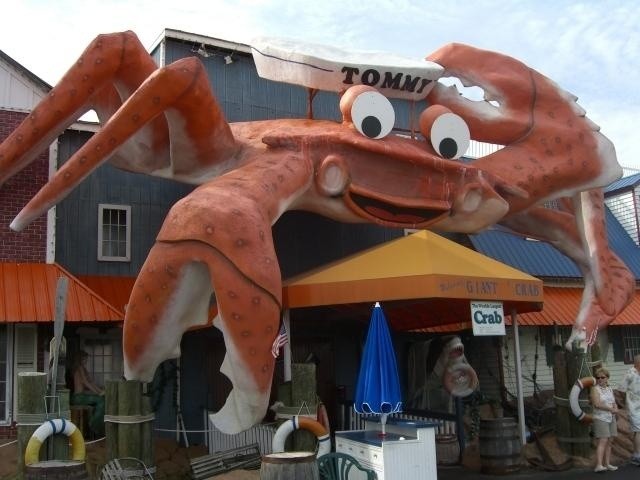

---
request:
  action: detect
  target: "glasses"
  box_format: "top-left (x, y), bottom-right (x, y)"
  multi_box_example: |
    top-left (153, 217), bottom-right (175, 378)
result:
top-left (596, 377), bottom-right (608, 379)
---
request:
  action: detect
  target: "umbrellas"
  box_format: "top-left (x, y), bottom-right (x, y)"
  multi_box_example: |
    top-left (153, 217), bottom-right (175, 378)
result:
top-left (353, 301), bottom-right (404, 436)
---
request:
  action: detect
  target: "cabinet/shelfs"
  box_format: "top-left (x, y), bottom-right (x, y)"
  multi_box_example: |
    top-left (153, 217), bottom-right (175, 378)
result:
top-left (335, 428), bottom-right (440, 479)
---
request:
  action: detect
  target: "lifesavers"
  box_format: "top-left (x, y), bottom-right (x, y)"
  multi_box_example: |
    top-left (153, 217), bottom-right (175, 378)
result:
top-left (272, 416), bottom-right (332, 466)
top-left (569, 377), bottom-right (598, 423)
top-left (24, 418), bottom-right (86, 466)
top-left (442, 363), bottom-right (478, 397)
top-left (319, 404), bottom-right (331, 439)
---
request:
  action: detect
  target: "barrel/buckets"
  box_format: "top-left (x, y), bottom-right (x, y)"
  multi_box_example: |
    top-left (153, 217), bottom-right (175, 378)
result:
top-left (24, 460), bottom-right (90, 480)
top-left (436, 433), bottom-right (460, 465)
top-left (478, 417), bottom-right (521, 475)
top-left (258, 451), bottom-right (321, 480)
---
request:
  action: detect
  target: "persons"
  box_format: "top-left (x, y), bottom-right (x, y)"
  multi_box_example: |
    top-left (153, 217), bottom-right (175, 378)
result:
top-left (69, 350), bottom-right (105, 436)
top-left (616, 355), bottom-right (640, 466)
top-left (589, 367), bottom-right (618, 473)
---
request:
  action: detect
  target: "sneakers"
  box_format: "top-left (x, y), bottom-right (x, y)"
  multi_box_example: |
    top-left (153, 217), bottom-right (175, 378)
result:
top-left (630, 455), bottom-right (640, 465)
top-left (604, 465), bottom-right (618, 470)
top-left (594, 466), bottom-right (607, 472)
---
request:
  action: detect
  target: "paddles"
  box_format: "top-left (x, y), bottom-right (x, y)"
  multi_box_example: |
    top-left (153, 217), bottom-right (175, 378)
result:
top-left (48, 277), bottom-right (68, 459)
